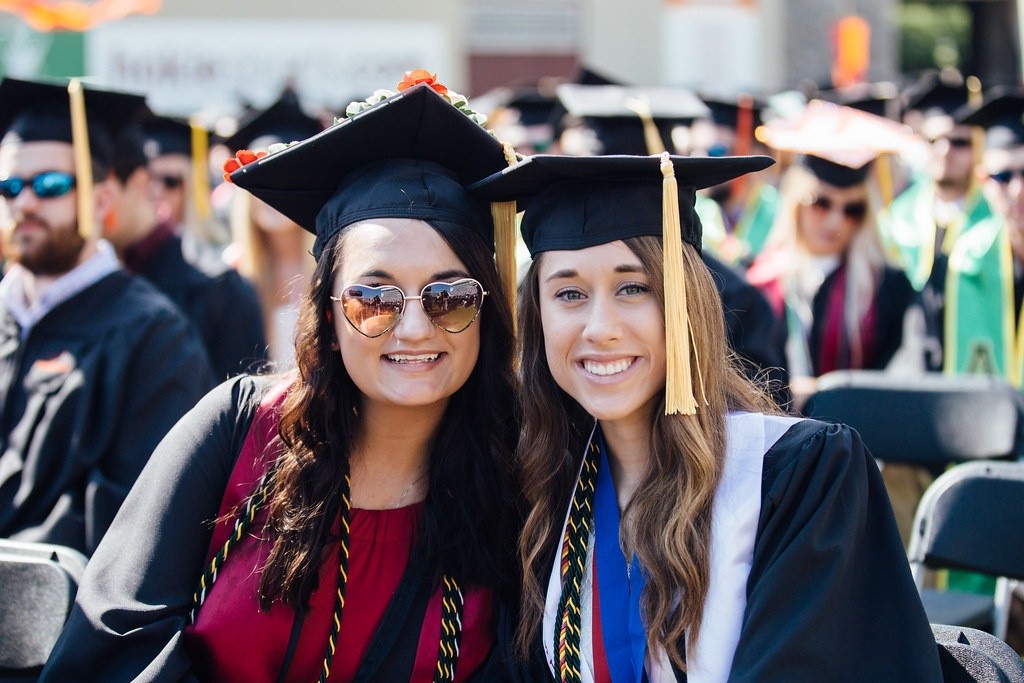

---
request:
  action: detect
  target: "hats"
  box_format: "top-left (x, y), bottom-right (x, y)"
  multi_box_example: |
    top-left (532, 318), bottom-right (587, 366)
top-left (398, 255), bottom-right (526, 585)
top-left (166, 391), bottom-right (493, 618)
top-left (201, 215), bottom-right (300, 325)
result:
top-left (466, 152), bottom-right (777, 417)
top-left (151, 102), bottom-right (317, 152)
top-left (230, 84), bottom-right (533, 381)
top-left (0, 78), bottom-right (146, 240)
top-left (484, 69), bottom-right (1024, 219)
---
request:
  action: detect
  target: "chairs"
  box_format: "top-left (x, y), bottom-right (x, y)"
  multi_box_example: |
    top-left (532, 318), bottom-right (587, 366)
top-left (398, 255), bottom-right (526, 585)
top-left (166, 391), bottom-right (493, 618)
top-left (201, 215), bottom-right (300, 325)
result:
top-left (1, 542), bottom-right (90, 677)
top-left (797, 367), bottom-right (1023, 683)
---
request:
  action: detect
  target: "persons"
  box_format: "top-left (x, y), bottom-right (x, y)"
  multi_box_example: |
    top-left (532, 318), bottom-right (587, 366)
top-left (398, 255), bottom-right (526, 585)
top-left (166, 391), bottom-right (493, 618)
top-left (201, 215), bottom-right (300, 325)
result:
top-left (0, 72), bottom-right (1024, 601)
top-left (36, 85), bottom-right (547, 683)
top-left (472, 152), bottom-right (945, 683)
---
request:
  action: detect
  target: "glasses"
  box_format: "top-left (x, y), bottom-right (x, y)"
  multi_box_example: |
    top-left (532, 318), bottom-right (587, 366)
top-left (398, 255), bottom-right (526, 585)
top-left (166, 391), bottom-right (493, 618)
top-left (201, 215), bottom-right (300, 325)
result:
top-left (152, 173), bottom-right (184, 192)
top-left (925, 137), bottom-right (971, 148)
top-left (987, 169), bottom-right (1024, 183)
top-left (0, 173), bottom-right (76, 202)
top-left (329, 277), bottom-right (489, 338)
top-left (803, 194), bottom-right (866, 223)
